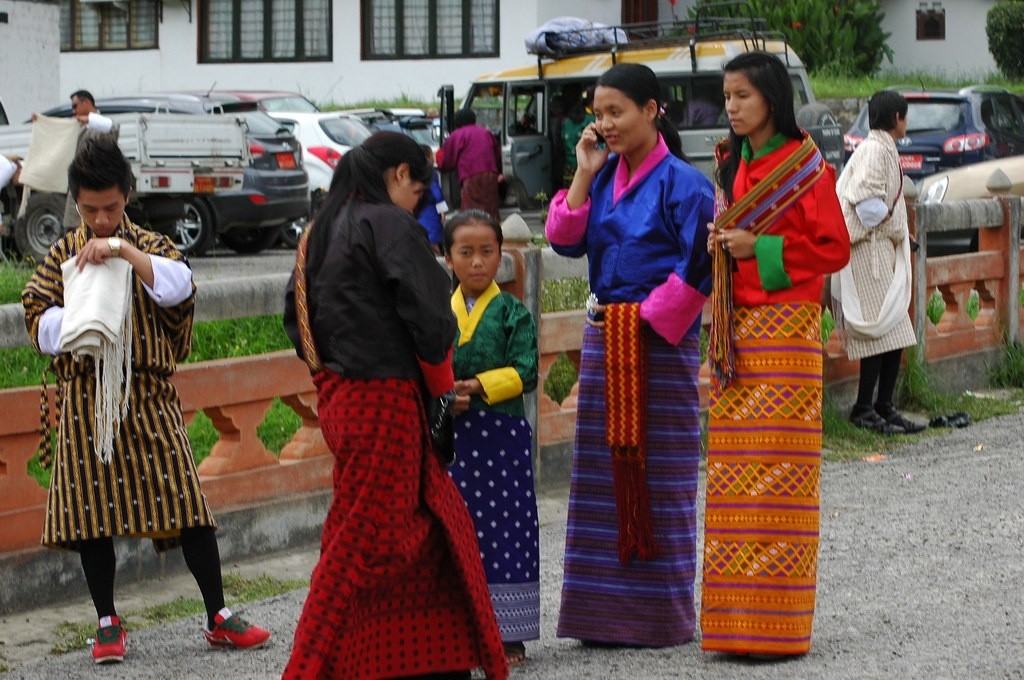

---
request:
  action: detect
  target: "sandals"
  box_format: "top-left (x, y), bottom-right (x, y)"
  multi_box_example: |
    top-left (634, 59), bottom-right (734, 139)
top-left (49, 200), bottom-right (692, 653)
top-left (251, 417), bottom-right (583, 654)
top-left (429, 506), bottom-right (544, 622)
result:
top-left (503, 641), bottom-right (525, 664)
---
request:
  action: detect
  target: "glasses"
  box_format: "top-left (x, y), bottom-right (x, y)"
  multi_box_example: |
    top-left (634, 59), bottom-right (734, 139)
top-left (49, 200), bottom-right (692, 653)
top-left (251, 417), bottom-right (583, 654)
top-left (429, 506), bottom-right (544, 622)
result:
top-left (71, 101), bottom-right (82, 110)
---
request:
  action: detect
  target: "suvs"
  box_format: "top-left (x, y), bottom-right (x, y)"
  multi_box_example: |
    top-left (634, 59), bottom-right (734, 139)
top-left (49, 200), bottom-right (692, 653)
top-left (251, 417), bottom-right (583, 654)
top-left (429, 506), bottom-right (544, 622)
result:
top-left (843, 83), bottom-right (1024, 184)
top-left (41, 93), bottom-right (310, 257)
top-left (432, 2), bottom-right (847, 210)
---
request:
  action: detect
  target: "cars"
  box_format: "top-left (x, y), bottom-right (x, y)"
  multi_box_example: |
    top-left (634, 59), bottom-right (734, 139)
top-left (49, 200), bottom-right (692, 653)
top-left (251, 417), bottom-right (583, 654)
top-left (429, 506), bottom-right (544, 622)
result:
top-left (915, 156), bottom-right (1024, 253)
top-left (232, 93), bottom-right (447, 249)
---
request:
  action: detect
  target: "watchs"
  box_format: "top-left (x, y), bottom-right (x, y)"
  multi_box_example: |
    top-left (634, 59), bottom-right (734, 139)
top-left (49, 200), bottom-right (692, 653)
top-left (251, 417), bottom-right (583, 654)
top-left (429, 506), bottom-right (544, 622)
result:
top-left (108, 237), bottom-right (120, 257)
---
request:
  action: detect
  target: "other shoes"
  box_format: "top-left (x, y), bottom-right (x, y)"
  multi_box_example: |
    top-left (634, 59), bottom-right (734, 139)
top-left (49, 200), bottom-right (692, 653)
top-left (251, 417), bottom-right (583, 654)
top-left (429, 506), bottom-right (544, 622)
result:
top-left (848, 404), bottom-right (904, 433)
top-left (874, 402), bottom-right (927, 431)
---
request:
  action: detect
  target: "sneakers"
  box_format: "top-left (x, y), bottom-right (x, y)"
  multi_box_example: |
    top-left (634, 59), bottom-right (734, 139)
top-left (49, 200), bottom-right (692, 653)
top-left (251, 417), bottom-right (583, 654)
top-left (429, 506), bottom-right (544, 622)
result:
top-left (92, 615), bottom-right (128, 663)
top-left (205, 607), bottom-right (271, 649)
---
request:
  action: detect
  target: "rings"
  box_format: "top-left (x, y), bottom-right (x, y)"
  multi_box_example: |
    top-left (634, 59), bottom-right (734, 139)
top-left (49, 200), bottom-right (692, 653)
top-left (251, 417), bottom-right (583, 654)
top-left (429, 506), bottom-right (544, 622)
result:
top-left (722, 243), bottom-right (726, 249)
top-left (722, 233), bottom-right (725, 241)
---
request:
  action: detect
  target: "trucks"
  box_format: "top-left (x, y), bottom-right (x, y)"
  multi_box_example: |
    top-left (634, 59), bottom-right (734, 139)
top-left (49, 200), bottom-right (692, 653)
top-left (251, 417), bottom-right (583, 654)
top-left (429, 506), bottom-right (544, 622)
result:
top-left (0, 95), bottom-right (248, 264)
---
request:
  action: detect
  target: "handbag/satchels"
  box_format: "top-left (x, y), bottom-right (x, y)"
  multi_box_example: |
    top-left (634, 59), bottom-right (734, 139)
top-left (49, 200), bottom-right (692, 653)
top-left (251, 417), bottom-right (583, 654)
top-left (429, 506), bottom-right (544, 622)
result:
top-left (525, 17), bottom-right (627, 52)
top-left (421, 385), bottom-right (455, 465)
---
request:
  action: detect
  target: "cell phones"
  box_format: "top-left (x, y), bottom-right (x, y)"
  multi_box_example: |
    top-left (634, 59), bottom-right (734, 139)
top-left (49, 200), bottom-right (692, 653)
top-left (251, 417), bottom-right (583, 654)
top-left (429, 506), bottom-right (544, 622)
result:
top-left (595, 130), bottom-right (605, 149)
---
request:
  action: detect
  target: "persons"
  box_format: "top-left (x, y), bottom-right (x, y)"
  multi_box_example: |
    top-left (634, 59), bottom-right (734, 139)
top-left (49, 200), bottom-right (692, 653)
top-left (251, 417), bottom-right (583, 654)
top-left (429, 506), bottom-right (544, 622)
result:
top-left (0, 152), bottom-right (22, 264)
top-left (436, 108), bottom-right (504, 223)
top-left (545, 63), bottom-right (716, 647)
top-left (831, 91), bottom-right (926, 436)
top-left (31, 89), bottom-right (120, 233)
top-left (699, 51), bottom-right (851, 655)
top-left (666, 98), bottom-right (720, 129)
top-left (565, 85), bottom-right (605, 158)
top-left (20, 132), bottom-right (272, 664)
top-left (282, 130), bottom-right (541, 680)
